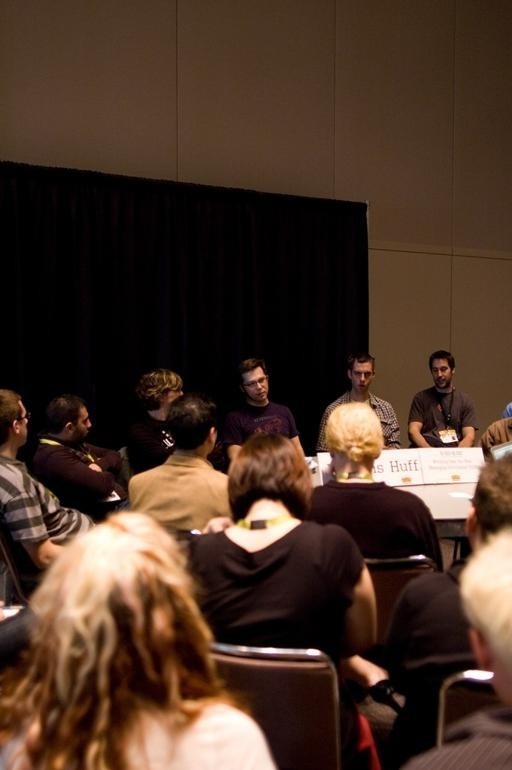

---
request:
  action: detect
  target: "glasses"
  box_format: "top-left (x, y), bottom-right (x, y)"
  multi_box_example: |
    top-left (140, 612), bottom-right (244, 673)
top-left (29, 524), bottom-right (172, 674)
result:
top-left (243, 376), bottom-right (266, 387)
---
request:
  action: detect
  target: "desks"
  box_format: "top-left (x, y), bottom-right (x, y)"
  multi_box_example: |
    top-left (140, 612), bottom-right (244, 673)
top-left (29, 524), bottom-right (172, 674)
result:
top-left (381, 478), bottom-right (476, 574)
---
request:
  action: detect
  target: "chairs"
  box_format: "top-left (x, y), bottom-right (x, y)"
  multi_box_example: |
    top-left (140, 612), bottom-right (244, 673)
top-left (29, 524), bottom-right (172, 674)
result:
top-left (438, 669), bottom-right (495, 741)
top-left (207, 642), bottom-right (341, 770)
top-left (363, 556), bottom-right (437, 647)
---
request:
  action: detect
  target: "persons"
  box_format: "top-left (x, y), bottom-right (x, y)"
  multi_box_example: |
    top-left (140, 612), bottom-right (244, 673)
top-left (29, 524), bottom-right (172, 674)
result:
top-left (30, 390), bottom-right (132, 518)
top-left (125, 366), bottom-right (188, 477)
top-left (181, 427), bottom-right (407, 769)
top-left (388, 452), bottom-right (512, 699)
top-left (300, 401), bottom-right (445, 577)
top-left (1, 387), bottom-right (101, 569)
top-left (316, 355), bottom-right (402, 455)
top-left (223, 354), bottom-right (307, 473)
top-left (479, 401), bottom-right (512, 461)
top-left (129, 394), bottom-right (232, 544)
top-left (0, 509), bottom-right (276, 770)
top-left (396, 525), bottom-right (512, 768)
top-left (407, 351), bottom-right (479, 451)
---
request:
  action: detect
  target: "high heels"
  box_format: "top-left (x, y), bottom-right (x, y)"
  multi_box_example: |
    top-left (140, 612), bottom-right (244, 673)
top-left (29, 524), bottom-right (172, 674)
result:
top-left (365, 679), bottom-right (404, 716)
